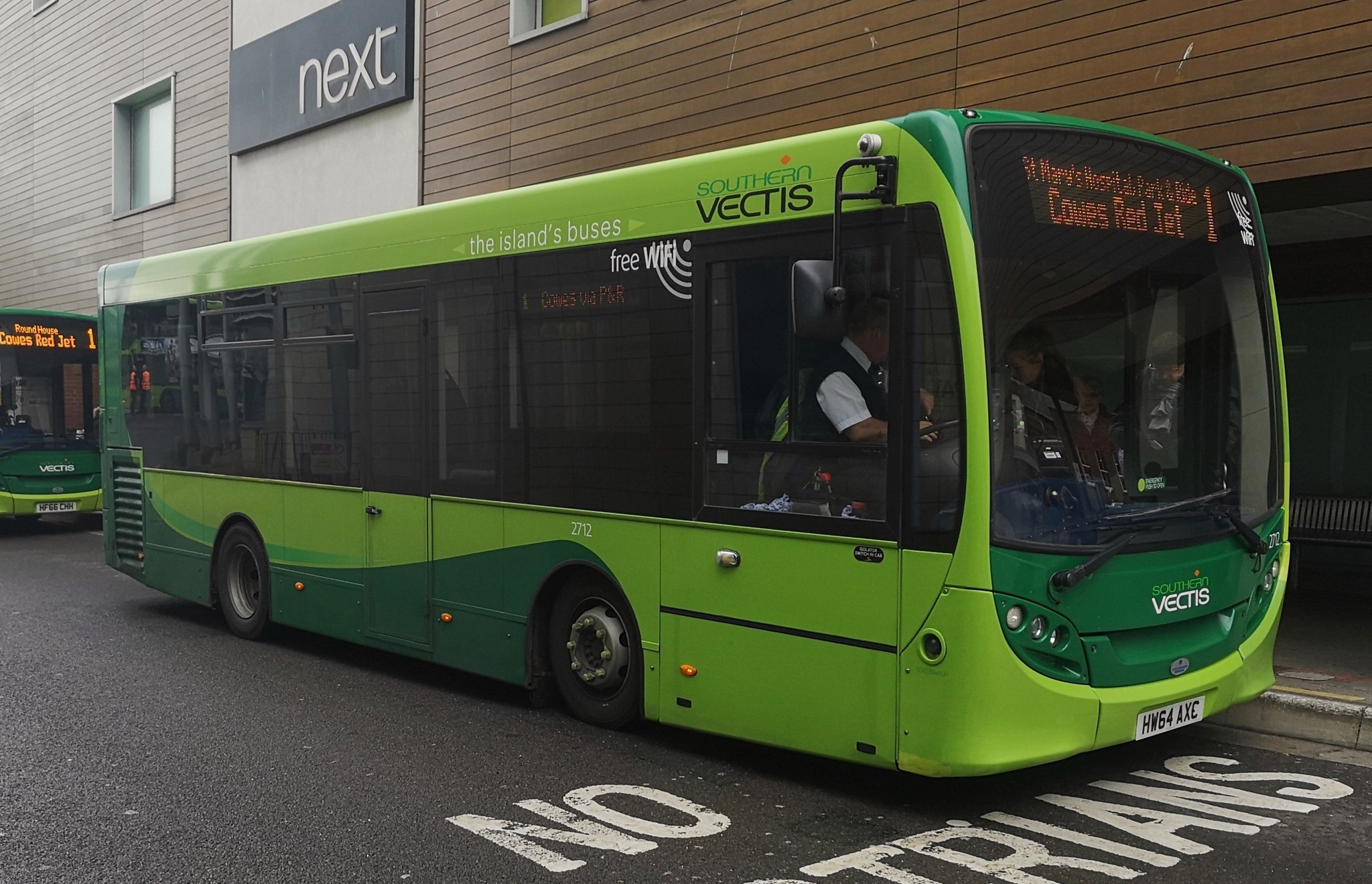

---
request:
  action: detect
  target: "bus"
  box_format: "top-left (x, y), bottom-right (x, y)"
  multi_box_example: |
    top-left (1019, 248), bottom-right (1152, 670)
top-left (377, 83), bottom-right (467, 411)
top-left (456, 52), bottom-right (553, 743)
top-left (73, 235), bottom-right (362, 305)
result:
top-left (0, 306), bottom-right (100, 524)
top-left (98, 111), bottom-right (1290, 780)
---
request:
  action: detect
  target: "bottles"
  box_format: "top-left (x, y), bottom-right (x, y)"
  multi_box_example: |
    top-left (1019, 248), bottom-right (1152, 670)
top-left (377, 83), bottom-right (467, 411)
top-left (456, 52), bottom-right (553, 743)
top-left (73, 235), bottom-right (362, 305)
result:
top-left (851, 502), bottom-right (870, 520)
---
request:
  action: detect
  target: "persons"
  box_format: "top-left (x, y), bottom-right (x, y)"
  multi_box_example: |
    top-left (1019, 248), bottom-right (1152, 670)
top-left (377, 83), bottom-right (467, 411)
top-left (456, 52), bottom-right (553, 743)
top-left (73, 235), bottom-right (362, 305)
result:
top-left (803, 287), bottom-right (934, 535)
top-left (1002, 330), bottom-right (1210, 502)
